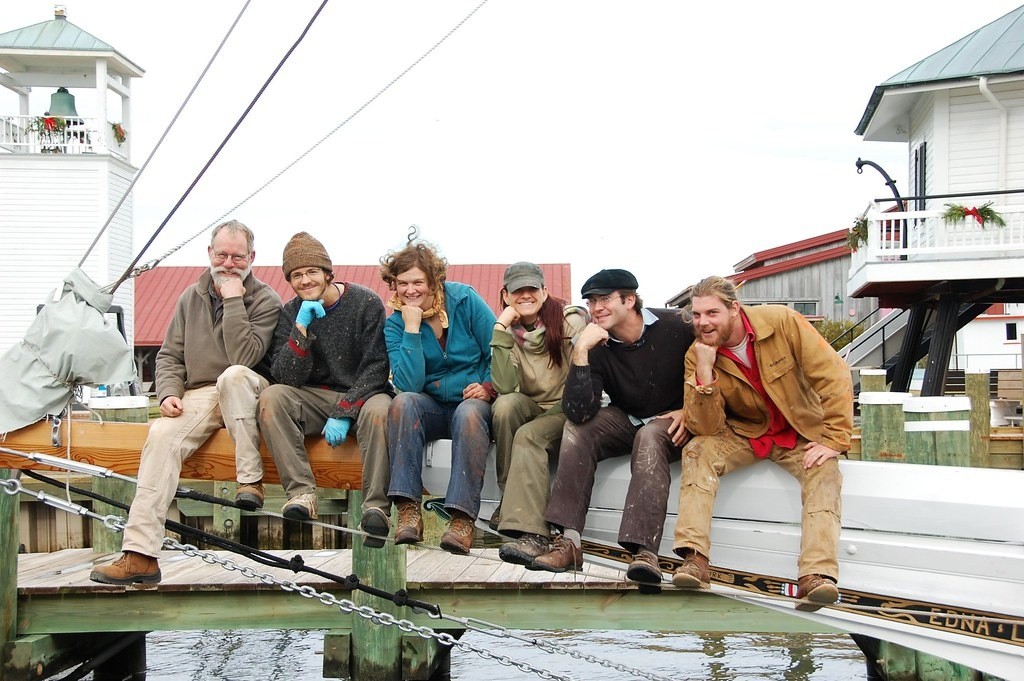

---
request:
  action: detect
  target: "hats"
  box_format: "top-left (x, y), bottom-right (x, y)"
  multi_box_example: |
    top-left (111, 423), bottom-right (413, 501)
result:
top-left (503, 261), bottom-right (544, 293)
top-left (282, 231), bottom-right (333, 282)
top-left (581, 268), bottom-right (638, 299)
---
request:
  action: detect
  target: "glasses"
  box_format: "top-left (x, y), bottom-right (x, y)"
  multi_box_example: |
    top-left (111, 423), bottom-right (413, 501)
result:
top-left (586, 295), bottom-right (626, 307)
top-left (212, 250), bottom-right (249, 264)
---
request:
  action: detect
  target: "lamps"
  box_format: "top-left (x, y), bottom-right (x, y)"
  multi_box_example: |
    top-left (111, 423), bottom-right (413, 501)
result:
top-left (834, 292), bottom-right (844, 305)
top-left (896, 125), bottom-right (907, 136)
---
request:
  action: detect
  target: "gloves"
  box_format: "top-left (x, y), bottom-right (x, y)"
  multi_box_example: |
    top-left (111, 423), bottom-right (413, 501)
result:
top-left (321, 417), bottom-right (351, 449)
top-left (294, 299), bottom-right (326, 328)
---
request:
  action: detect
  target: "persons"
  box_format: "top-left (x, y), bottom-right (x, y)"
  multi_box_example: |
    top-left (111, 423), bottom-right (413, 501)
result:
top-left (670, 276), bottom-right (853, 612)
top-left (259, 230), bottom-right (397, 548)
top-left (90, 219), bottom-right (283, 586)
top-left (384, 226), bottom-right (497, 554)
top-left (489, 261), bottom-right (591, 566)
top-left (533, 270), bottom-right (694, 596)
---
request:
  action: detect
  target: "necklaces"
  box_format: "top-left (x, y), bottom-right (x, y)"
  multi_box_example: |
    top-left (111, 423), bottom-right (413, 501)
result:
top-left (312, 282), bottom-right (340, 312)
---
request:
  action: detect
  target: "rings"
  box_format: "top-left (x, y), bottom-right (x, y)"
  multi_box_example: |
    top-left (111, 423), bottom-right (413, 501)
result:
top-left (819, 452), bottom-right (822, 455)
top-left (469, 385), bottom-right (473, 389)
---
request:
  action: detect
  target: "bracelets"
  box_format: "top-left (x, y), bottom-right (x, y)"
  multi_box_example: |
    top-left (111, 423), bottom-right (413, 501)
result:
top-left (494, 322), bottom-right (506, 329)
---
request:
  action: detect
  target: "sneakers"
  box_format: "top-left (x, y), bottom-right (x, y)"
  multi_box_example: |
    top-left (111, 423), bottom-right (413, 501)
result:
top-left (671, 551), bottom-right (711, 590)
top-left (281, 494), bottom-right (318, 520)
top-left (627, 549), bottom-right (661, 594)
top-left (439, 510), bottom-right (473, 555)
top-left (532, 534), bottom-right (584, 584)
top-left (499, 531), bottom-right (548, 570)
top-left (90, 551), bottom-right (162, 584)
top-left (394, 501), bottom-right (424, 545)
top-left (235, 479), bottom-right (264, 512)
top-left (795, 574), bottom-right (838, 611)
top-left (361, 506), bottom-right (393, 550)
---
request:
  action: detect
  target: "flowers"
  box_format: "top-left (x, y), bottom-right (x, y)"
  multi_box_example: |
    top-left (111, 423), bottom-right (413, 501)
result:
top-left (23, 116), bottom-right (68, 141)
top-left (112, 122), bottom-right (128, 148)
